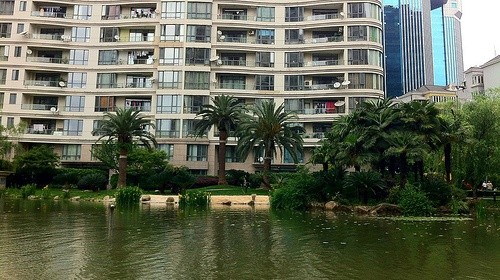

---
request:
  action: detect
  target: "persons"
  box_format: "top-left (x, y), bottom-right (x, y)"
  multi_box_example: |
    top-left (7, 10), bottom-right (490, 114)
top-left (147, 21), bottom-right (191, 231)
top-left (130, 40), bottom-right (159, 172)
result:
top-left (482, 180), bottom-right (487, 190)
top-left (486, 181), bottom-right (493, 191)
